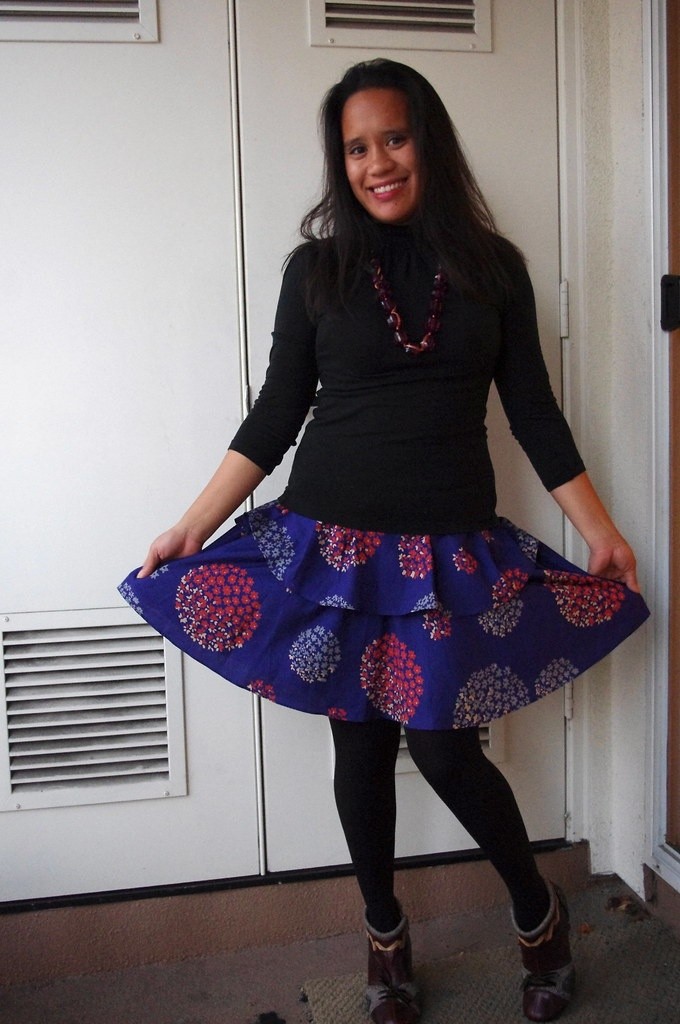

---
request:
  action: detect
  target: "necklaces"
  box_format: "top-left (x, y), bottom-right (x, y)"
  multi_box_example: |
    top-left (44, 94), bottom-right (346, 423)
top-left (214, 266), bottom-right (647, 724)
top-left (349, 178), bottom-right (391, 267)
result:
top-left (367, 234), bottom-right (448, 353)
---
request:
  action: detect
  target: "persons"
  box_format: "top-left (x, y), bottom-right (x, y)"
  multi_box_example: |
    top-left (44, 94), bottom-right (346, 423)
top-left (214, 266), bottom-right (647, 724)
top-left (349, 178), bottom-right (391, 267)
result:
top-left (118, 56), bottom-right (651, 1024)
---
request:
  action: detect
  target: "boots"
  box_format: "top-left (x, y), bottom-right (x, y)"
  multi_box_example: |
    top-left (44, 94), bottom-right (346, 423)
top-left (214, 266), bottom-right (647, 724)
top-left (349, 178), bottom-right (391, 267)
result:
top-left (509, 875), bottom-right (577, 1023)
top-left (362, 914), bottom-right (422, 1024)
